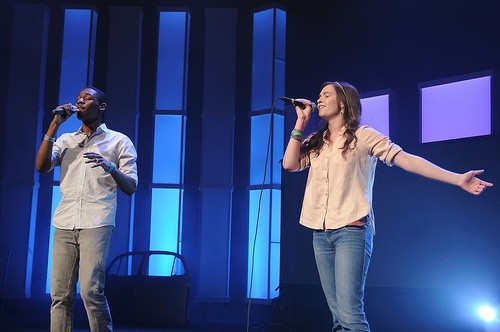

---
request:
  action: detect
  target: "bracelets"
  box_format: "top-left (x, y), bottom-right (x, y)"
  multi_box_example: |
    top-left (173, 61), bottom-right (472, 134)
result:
top-left (290, 129), bottom-right (303, 141)
top-left (44, 135), bottom-right (56, 142)
top-left (105, 162), bottom-right (117, 174)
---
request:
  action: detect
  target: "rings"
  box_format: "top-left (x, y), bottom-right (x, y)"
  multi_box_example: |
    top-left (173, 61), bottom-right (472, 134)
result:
top-left (96, 159), bottom-right (98, 163)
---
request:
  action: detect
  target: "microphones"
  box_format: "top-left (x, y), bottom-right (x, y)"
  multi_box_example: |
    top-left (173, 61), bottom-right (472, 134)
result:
top-left (280, 96), bottom-right (316, 112)
top-left (51, 106), bottom-right (77, 115)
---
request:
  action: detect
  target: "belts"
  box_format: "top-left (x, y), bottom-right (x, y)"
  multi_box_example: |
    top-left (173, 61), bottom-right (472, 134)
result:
top-left (347, 221), bottom-right (365, 227)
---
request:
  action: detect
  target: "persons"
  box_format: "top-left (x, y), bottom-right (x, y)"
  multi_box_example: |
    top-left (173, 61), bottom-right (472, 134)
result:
top-left (282, 80), bottom-right (493, 332)
top-left (35, 86), bottom-right (139, 332)
